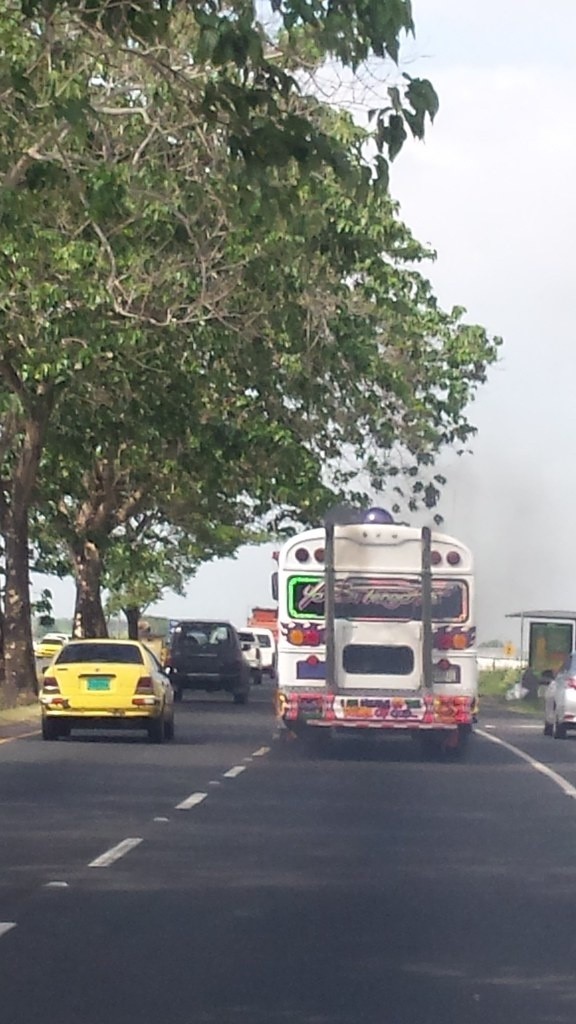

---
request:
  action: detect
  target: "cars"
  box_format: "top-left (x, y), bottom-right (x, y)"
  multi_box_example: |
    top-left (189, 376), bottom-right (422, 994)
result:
top-left (38, 638), bottom-right (175, 740)
top-left (34, 631), bottom-right (66, 660)
top-left (543, 654), bottom-right (576, 739)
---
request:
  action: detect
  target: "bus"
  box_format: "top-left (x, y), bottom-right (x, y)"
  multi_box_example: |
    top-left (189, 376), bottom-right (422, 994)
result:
top-left (273, 521), bottom-right (476, 761)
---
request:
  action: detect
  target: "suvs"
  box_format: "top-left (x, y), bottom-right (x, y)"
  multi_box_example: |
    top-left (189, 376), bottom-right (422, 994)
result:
top-left (162, 620), bottom-right (250, 705)
top-left (233, 627), bottom-right (275, 686)
